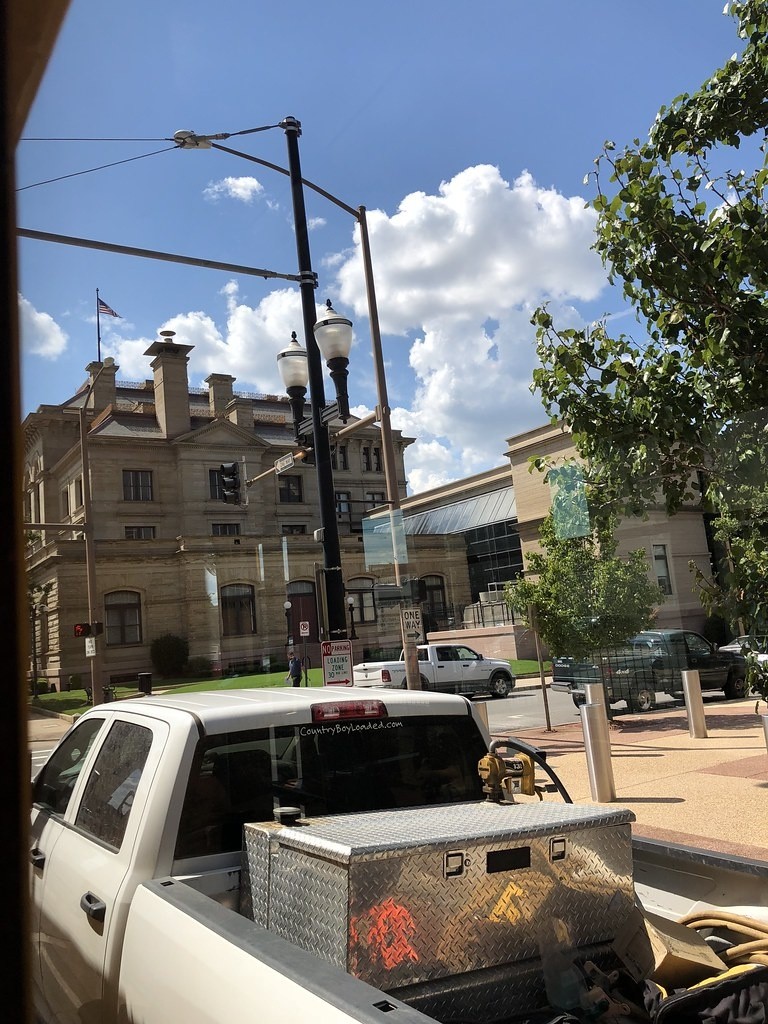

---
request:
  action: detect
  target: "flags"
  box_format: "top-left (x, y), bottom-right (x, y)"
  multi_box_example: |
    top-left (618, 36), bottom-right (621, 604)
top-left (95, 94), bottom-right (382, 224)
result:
top-left (98, 298), bottom-right (122, 318)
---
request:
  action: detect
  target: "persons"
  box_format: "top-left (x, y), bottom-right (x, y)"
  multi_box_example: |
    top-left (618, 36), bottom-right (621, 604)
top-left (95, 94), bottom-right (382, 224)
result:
top-left (287, 652), bottom-right (308, 687)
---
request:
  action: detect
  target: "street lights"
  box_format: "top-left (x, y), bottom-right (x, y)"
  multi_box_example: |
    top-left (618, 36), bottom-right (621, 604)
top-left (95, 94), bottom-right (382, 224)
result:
top-left (77, 356), bottom-right (114, 708)
top-left (177, 125), bottom-right (423, 688)
top-left (276, 293), bottom-right (355, 686)
top-left (282, 601), bottom-right (296, 647)
top-left (345, 594), bottom-right (361, 641)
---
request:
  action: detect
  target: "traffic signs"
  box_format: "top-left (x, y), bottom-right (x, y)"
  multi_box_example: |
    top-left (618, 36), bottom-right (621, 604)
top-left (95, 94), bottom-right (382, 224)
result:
top-left (321, 639), bottom-right (354, 688)
top-left (401, 607), bottom-right (426, 645)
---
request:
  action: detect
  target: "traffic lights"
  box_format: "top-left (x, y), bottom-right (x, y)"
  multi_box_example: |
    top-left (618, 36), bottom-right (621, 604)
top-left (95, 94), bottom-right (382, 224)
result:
top-left (407, 577), bottom-right (427, 604)
top-left (74, 622), bottom-right (90, 638)
top-left (220, 461), bottom-right (242, 508)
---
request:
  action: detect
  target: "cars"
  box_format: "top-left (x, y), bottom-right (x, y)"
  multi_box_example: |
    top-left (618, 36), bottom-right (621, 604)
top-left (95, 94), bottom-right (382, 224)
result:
top-left (718, 634), bottom-right (768, 668)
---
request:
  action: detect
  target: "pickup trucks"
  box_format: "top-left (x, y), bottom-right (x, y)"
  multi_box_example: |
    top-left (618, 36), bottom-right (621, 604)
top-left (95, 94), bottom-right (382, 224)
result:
top-left (29, 688), bottom-right (768, 1024)
top-left (551, 629), bottom-right (751, 714)
top-left (355, 644), bottom-right (519, 700)
